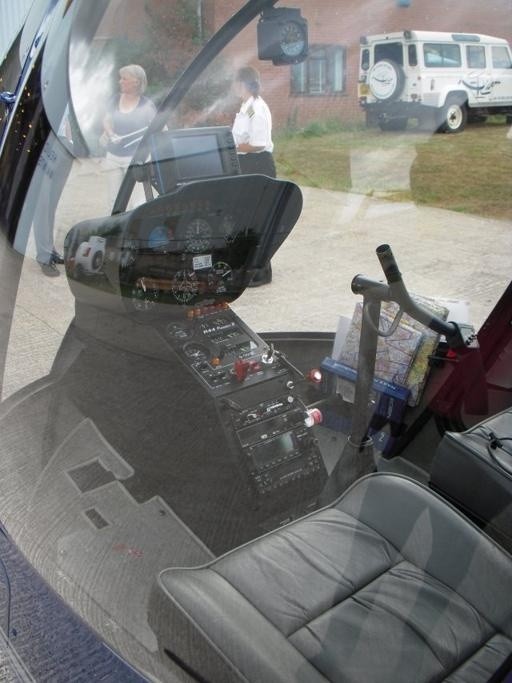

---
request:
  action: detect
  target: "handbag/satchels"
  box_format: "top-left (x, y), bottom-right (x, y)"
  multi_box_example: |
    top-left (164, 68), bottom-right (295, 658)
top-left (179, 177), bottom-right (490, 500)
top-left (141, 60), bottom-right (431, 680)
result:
top-left (98, 94), bottom-right (120, 150)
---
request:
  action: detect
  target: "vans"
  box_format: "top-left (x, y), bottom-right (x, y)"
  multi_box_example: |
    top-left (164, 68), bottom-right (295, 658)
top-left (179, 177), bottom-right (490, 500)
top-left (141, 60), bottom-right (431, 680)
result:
top-left (352, 25), bottom-right (512, 129)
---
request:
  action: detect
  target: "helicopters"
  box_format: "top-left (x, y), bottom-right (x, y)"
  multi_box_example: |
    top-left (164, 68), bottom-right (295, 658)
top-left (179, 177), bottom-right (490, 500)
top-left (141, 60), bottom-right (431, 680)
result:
top-left (0, 0), bottom-right (512, 683)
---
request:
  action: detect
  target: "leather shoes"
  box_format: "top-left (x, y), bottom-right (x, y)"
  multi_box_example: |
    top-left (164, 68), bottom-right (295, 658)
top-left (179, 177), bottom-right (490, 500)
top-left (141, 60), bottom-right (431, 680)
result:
top-left (51, 249), bottom-right (65, 264)
top-left (36, 256), bottom-right (60, 277)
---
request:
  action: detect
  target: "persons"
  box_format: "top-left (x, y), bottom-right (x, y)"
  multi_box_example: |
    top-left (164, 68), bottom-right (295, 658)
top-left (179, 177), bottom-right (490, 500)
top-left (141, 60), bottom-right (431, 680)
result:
top-left (30, 62), bottom-right (73, 278)
top-left (295, 317), bottom-right (484, 682)
top-left (96, 61), bottom-right (169, 218)
top-left (227, 64), bottom-right (276, 287)
